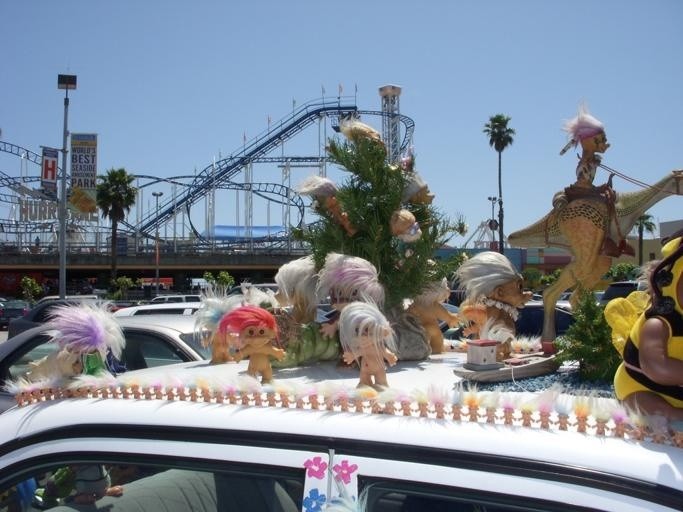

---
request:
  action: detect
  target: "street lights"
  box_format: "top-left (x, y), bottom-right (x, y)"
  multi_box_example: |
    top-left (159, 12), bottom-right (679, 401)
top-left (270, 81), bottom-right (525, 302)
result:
top-left (54, 71), bottom-right (76, 299)
top-left (152, 192), bottom-right (162, 298)
top-left (487, 196), bottom-right (497, 251)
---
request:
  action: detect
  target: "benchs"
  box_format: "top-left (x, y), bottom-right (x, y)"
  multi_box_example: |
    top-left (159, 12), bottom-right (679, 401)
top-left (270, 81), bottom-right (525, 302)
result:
top-left (44, 465), bottom-right (299, 512)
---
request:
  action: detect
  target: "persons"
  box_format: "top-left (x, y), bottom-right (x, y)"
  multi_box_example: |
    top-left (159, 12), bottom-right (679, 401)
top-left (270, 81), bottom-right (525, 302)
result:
top-left (605, 234), bottom-right (682, 441)
top-left (192, 288), bottom-right (279, 362)
top-left (46, 300), bottom-right (127, 369)
top-left (451, 251), bottom-right (532, 357)
top-left (407, 259), bottom-right (457, 354)
top-left (6, 369), bottom-right (683, 448)
top-left (217, 305), bottom-right (287, 385)
top-left (316, 253), bottom-right (398, 389)
top-left (274, 256), bottom-right (322, 322)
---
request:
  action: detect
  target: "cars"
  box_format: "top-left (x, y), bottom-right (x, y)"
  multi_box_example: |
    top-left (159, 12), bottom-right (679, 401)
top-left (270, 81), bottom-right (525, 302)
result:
top-left (0, 343), bottom-right (682, 511)
